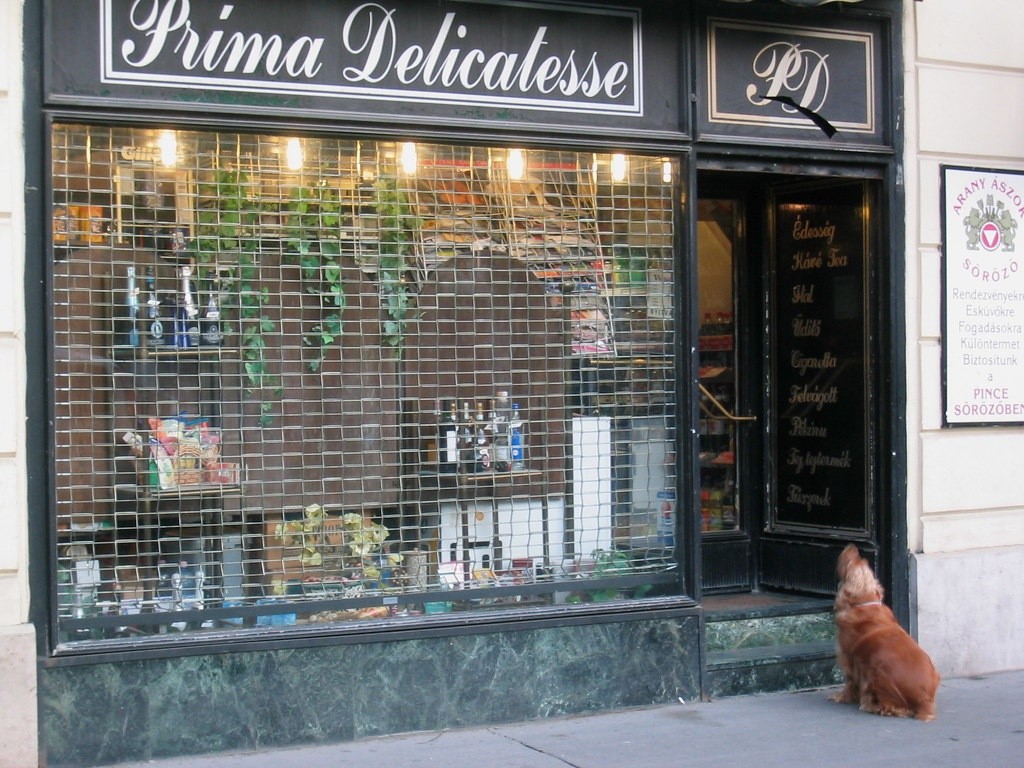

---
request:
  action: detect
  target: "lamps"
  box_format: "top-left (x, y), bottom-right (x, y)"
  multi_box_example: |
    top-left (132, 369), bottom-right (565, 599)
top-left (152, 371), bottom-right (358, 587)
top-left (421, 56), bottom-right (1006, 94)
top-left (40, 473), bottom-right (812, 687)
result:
top-left (507, 148), bottom-right (526, 181)
top-left (285, 136), bottom-right (304, 171)
top-left (402, 142), bottom-right (419, 175)
top-left (158, 128), bottom-right (178, 167)
top-left (663, 162), bottom-right (672, 182)
top-left (611, 154), bottom-right (627, 181)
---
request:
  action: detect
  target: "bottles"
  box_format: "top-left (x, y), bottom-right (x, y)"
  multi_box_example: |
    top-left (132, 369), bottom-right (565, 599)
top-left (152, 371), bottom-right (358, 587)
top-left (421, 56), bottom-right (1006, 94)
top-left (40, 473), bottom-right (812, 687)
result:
top-left (114, 267), bottom-right (143, 360)
top-left (700, 388), bottom-right (731, 454)
top-left (699, 312), bottom-right (735, 368)
top-left (478, 555), bottom-right (496, 605)
top-left (174, 266), bottom-right (200, 349)
top-left (172, 570), bottom-right (183, 611)
top-left (176, 561), bottom-right (195, 610)
top-left (155, 559), bottom-right (172, 613)
top-left (697, 480), bottom-right (735, 531)
top-left (146, 266), bottom-right (165, 347)
top-left (427, 391), bottom-right (526, 474)
top-left (200, 270), bottom-right (225, 346)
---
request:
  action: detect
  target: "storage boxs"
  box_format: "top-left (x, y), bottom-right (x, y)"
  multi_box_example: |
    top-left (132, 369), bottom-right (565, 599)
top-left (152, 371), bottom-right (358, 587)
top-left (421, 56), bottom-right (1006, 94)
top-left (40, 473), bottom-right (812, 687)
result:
top-left (424, 601), bottom-right (453, 615)
top-left (210, 463), bottom-right (241, 484)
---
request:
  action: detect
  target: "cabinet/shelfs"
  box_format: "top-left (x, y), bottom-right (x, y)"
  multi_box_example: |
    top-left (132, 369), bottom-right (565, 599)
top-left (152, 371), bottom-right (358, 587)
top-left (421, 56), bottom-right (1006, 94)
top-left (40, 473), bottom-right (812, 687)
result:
top-left (699, 350), bottom-right (737, 532)
top-left (103, 270), bottom-right (252, 600)
top-left (398, 394), bottom-right (553, 611)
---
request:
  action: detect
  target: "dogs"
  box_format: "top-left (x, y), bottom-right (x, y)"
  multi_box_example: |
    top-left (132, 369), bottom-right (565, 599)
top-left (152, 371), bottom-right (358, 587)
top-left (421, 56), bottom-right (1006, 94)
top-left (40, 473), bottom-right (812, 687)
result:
top-left (834, 544), bottom-right (940, 723)
